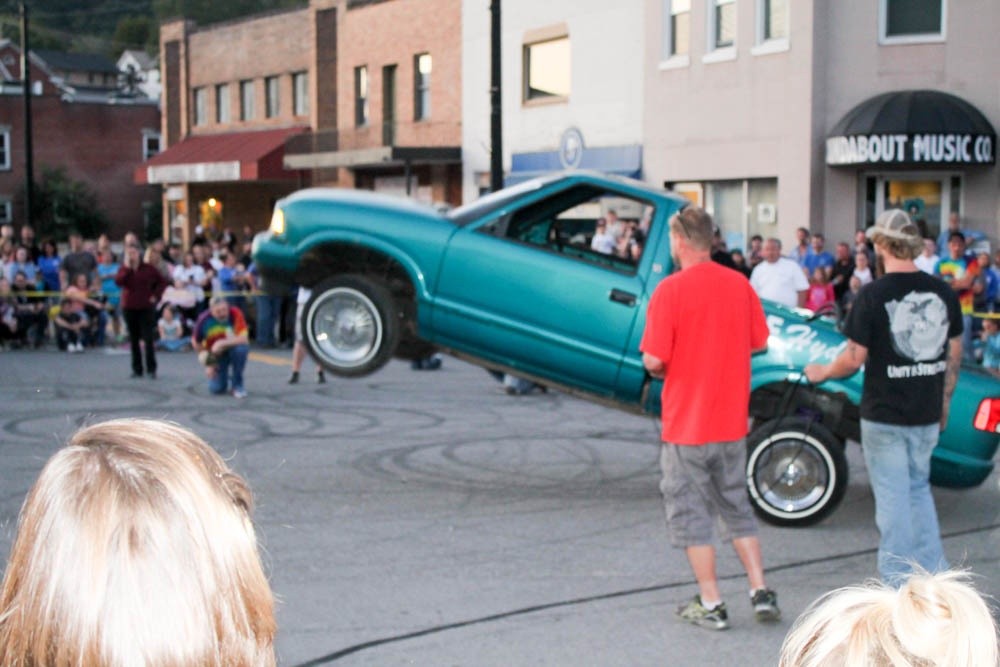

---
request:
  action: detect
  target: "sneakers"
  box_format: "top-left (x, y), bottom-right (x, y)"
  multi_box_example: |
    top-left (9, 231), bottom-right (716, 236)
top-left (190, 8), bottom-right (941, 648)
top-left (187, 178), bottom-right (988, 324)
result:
top-left (673, 594), bottom-right (729, 630)
top-left (751, 589), bottom-right (782, 622)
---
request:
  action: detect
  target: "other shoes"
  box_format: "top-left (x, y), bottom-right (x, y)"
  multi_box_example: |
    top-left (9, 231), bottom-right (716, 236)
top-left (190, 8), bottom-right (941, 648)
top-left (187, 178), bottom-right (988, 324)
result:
top-left (232, 389), bottom-right (247, 399)
top-left (288, 373), bottom-right (299, 383)
top-left (411, 361), bottom-right (421, 369)
top-left (315, 372), bottom-right (325, 383)
top-left (76, 343), bottom-right (84, 352)
top-left (68, 343), bottom-right (75, 353)
top-left (131, 373), bottom-right (142, 377)
top-left (149, 373), bottom-right (155, 378)
top-left (520, 390), bottom-right (532, 394)
top-left (422, 358), bottom-right (441, 369)
top-left (507, 386), bottom-right (516, 394)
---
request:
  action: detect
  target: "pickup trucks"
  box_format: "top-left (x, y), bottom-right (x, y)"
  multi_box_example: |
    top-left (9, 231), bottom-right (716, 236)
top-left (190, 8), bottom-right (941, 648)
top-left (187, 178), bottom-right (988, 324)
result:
top-left (250, 166), bottom-right (999, 531)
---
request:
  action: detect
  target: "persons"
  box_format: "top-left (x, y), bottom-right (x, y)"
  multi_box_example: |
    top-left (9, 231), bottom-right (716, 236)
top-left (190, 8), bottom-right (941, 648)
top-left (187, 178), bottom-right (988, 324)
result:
top-left (802, 211), bottom-right (964, 579)
top-left (779, 556), bottom-right (998, 667)
top-left (411, 356), bottom-right (443, 370)
top-left (711, 224), bottom-right (1000, 378)
top-left (640, 202), bottom-right (781, 633)
top-left (502, 372), bottom-right (548, 394)
top-left (0, 224), bottom-right (295, 354)
top-left (592, 211), bottom-right (645, 261)
top-left (191, 296), bottom-right (250, 399)
top-left (116, 244), bottom-right (166, 378)
top-left (0, 418), bottom-right (280, 667)
top-left (289, 286), bottom-right (327, 384)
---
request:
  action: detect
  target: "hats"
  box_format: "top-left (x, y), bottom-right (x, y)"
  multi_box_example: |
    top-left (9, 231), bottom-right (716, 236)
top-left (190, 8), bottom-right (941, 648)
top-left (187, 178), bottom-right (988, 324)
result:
top-left (865, 209), bottom-right (920, 240)
top-left (948, 232), bottom-right (965, 242)
